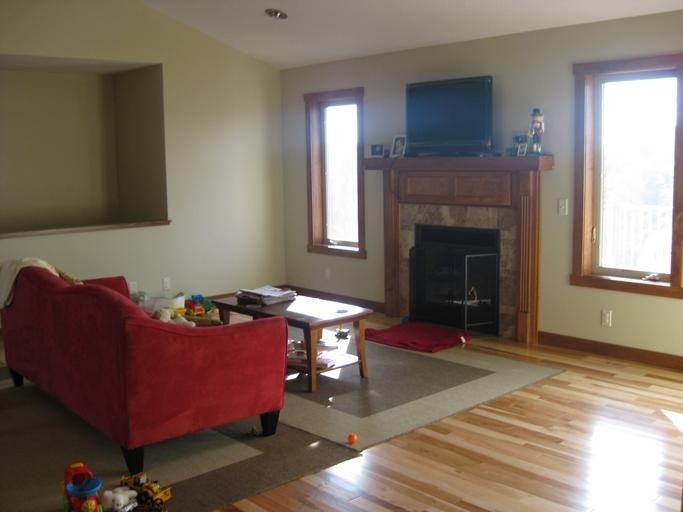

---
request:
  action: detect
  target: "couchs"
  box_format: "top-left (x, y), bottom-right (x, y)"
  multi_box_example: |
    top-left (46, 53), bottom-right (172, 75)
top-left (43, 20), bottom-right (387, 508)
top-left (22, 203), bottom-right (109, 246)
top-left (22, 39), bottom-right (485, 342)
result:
top-left (1, 256), bottom-right (288, 477)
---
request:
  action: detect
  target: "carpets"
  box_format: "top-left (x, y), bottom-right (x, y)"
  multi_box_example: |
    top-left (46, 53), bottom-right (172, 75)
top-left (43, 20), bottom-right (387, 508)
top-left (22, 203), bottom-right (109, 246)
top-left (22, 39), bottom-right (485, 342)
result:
top-left (1, 367), bottom-right (362, 511)
top-left (207, 308), bottom-right (564, 452)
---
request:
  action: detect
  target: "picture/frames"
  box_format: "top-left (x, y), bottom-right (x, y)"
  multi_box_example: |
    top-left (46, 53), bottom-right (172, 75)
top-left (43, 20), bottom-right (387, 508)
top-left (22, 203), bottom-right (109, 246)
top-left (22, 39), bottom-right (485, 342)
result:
top-left (516, 143), bottom-right (527, 156)
top-left (369, 144), bottom-right (383, 158)
top-left (390, 135), bottom-right (406, 157)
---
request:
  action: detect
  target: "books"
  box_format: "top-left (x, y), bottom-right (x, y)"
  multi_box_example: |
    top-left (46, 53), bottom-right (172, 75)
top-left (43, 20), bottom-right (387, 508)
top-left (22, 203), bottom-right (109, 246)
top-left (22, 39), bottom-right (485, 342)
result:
top-left (287, 340), bottom-right (338, 370)
top-left (236, 284), bottom-right (297, 306)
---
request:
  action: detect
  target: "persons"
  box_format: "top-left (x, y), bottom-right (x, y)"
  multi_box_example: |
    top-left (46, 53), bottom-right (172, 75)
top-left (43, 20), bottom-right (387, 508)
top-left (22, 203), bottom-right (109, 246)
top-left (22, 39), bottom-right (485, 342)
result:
top-left (529, 107), bottom-right (545, 153)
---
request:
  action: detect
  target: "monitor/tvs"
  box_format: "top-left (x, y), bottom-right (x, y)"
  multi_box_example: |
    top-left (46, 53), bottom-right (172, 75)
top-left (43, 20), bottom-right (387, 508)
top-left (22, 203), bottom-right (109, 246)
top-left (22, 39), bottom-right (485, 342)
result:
top-left (404, 74), bottom-right (494, 158)
